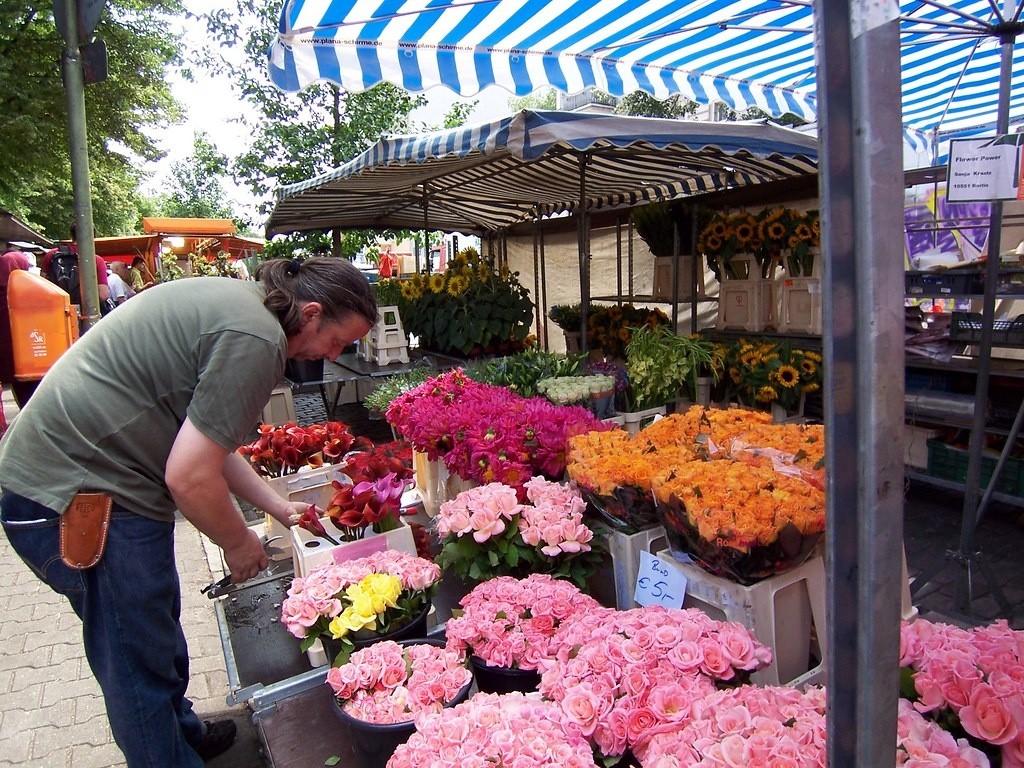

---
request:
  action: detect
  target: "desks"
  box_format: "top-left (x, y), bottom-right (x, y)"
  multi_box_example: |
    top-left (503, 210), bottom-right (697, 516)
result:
top-left (331, 351), bottom-right (466, 378)
top-left (284, 358), bottom-right (369, 422)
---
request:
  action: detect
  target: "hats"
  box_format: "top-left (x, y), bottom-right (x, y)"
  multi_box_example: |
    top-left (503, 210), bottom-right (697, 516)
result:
top-left (0, 252), bottom-right (30, 288)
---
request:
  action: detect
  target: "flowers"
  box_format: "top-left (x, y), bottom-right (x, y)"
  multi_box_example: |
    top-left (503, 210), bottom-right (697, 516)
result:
top-left (237, 303), bottom-right (1023, 768)
top-left (375, 245), bottom-right (534, 356)
top-left (696, 207), bottom-right (820, 282)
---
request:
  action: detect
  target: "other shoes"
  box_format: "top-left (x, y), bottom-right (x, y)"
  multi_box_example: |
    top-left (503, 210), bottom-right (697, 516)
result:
top-left (190, 719), bottom-right (237, 763)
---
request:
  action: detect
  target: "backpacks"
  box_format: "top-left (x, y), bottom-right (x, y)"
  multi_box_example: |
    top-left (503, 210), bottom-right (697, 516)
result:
top-left (49, 245), bottom-right (81, 304)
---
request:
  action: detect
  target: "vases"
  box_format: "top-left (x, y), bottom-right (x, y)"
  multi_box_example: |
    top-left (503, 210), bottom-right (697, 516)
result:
top-left (465, 648), bottom-right (542, 697)
top-left (371, 306), bottom-right (409, 366)
top-left (331, 639), bottom-right (474, 768)
top-left (439, 456), bottom-right (479, 506)
top-left (317, 598), bottom-right (431, 664)
top-left (676, 376), bottom-right (730, 412)
top-left (413, 441), bottom-right (438, 518)
top-left (780, 248), bottom-right (822, 336)
top-left (714, 252), bottom-right (778, 332)
top-left (499, 342), bottom-right (511, 356)
top-left (653, 549), bottom-right (827, 693)
top-left (511, 340), bottom-right (523, 353)
top-left (471, 347), bottom-right (482, 356)
top-left (289, 516), bottom-right (437, 668)
top-left (482, 346), bottom-right (495, 356)
top-left (616, 405), bottom-right (667, 434)
top-left (263, 463), bottom-right (357, 561)
top-left (738, 392), bottom-right (806, 424)
top-left (261, 385), bottom-right (299, 427)
top-left (589, 518), bottom-right (668, 613)
top-left (600, 415), bottom-right (626, 430)
top-left (356, 328), bottom-right (373, 362)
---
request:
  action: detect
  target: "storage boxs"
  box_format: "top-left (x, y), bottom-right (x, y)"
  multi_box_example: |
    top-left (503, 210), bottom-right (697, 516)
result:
top-left (951, 312), bottom-right (1024, 348)
top-left (925, 437), bottom-right (1023, 497)
top-left (904, 423), bottom-right (942, 472)
top-left (906, 257), bottom-right (1024, 294)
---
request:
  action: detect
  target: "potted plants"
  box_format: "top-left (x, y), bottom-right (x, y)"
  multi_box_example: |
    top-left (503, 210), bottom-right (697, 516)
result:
top-left (547, 304), bottom-right (604, 354)
top-left (630, 199), bottom-right (714, 300)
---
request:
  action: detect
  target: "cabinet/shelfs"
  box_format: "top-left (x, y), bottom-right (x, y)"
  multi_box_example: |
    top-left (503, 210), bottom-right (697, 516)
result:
top-left (904, 293), bottom-right (1024, 507)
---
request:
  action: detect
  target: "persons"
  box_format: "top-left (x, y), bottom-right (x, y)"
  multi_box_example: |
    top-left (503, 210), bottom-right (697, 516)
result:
top-left (0, 219), bottom-right (153, 409)
top-left (0, 255), bottom-right (377, 768)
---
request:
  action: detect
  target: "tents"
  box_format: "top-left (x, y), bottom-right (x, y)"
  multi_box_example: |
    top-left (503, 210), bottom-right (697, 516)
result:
top-left (264, 109), bottom-right (818, 351)
top-left (266, 0), bottom-right (1024, 768)
